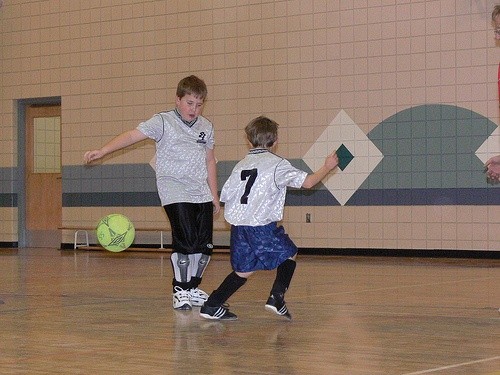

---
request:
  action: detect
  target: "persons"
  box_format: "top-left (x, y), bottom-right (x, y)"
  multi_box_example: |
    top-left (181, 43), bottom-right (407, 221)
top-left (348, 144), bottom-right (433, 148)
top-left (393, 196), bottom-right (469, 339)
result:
top-left (85, 76), bottom-right (221, 310)
top-left (199, 116), bottom-right (339, 322)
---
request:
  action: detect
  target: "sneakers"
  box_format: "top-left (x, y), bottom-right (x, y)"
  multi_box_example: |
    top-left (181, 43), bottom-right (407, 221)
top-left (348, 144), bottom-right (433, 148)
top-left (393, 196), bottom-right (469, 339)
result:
top-left (171, 286), bottom-right (193, 311)
top-left (199, 302), bottom-right (238, 320)
top-left (189, 288), bottom-right (209, 306)
top-left (264, 295), bottom-right (292, 320)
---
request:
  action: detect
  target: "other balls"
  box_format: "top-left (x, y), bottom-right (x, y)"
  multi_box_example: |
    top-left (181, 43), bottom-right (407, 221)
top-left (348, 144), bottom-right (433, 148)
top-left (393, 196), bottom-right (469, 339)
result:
top-left (96, 214), bottom-right (135, 252)
